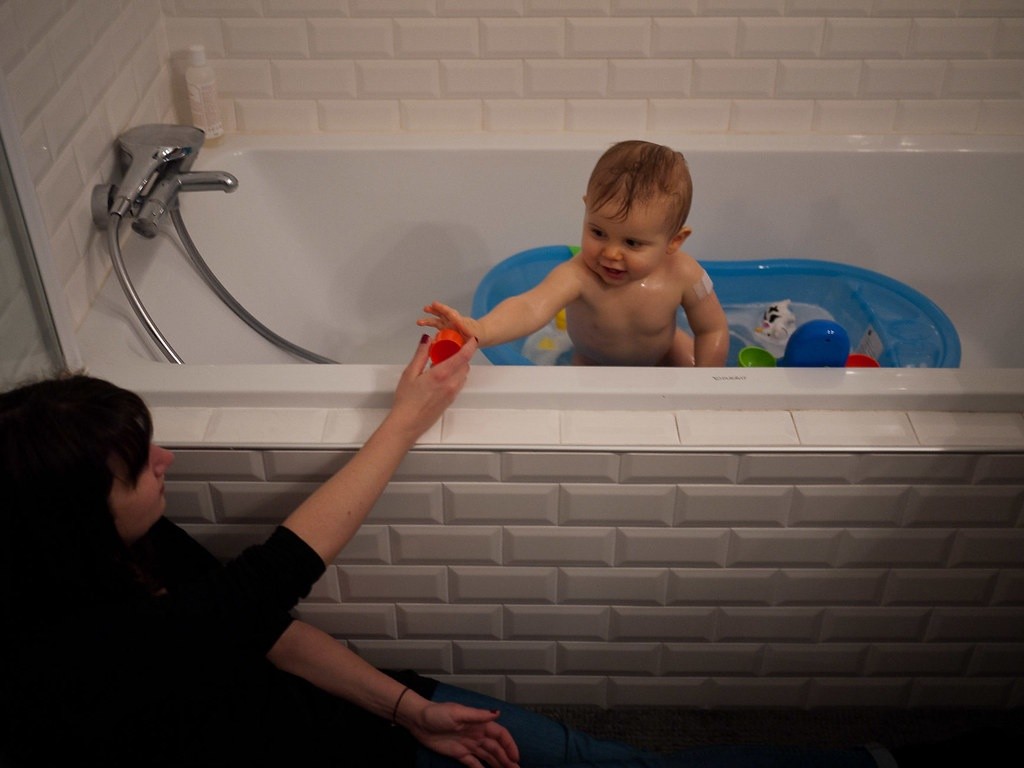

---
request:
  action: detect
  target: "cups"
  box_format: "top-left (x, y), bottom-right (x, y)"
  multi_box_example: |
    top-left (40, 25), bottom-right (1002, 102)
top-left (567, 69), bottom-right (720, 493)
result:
top-left (734, 345), bottom-right (776, 371)
top-left (427, 328), bottom-right (464, 367)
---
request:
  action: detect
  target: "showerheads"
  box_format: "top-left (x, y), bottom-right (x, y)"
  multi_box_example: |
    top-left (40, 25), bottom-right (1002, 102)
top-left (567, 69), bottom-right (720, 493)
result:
top-left (109, 122), bottom-right (206, 218)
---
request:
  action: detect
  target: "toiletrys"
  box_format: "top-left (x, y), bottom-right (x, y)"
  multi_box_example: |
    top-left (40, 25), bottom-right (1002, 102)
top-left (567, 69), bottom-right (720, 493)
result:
top-left (183, 44), bottom-right (225, 147)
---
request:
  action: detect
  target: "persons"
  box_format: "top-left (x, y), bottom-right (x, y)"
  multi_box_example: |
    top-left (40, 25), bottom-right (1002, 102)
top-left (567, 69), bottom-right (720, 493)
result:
top-left (0, 334), bottom-right (903, 768)
top-left (418, 140), bottom-right (729, 368)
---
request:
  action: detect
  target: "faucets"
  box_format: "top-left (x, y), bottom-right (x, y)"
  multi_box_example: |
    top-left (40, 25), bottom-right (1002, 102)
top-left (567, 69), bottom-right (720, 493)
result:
top-left (177, 166), bottom-right (239, 195)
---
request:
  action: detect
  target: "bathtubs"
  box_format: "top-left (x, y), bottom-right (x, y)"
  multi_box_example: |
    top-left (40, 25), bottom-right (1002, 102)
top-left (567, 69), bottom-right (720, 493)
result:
top-left (67, 107), bottom-right (1022, 724)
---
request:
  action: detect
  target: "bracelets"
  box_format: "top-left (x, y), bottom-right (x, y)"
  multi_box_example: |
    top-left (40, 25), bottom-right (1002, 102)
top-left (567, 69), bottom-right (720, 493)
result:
top-left (390, 686), bottom-right (415, 727)
